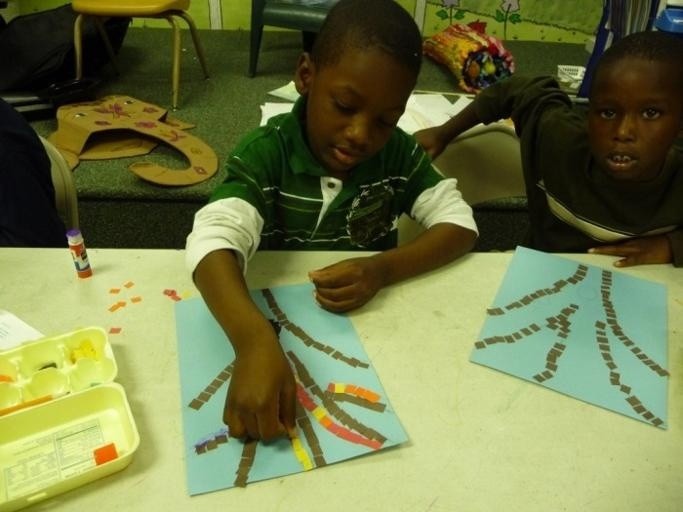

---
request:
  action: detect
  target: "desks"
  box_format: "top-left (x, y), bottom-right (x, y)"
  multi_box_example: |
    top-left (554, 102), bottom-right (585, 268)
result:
top-left (2, 247), bottom-right (683, 512)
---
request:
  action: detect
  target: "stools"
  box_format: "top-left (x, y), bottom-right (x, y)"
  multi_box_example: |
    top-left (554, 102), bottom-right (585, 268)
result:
top-left (71, 0), bottom-right (211, 111)
top-left (245, 0), bottom-right (329, 78)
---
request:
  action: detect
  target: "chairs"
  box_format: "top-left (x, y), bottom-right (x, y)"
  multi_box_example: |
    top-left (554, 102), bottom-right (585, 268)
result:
top-left (391, 118), bottom-right (529, 253)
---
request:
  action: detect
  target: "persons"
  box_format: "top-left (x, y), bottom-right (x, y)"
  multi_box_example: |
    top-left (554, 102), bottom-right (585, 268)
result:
top-left (412, 32), bottom-right (683, 268)
top-left (185, 0), bottom-right (480, 442)
